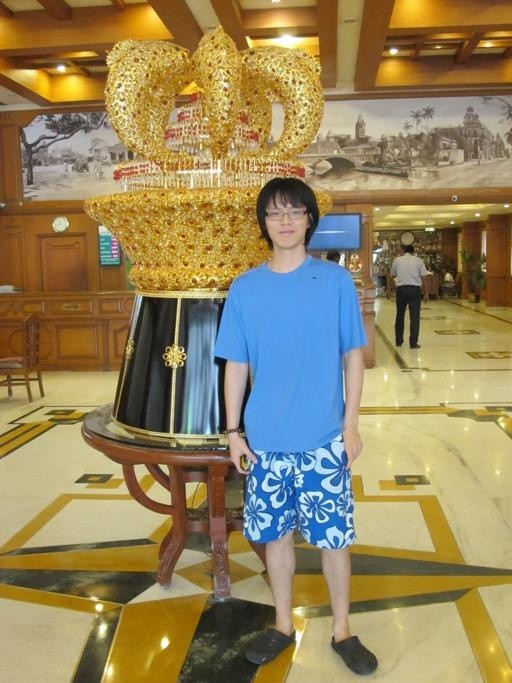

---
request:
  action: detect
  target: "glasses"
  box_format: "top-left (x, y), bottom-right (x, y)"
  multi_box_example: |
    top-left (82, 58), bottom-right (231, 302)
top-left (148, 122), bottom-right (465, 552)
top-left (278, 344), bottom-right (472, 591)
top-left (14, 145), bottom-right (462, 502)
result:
top-left (264, 209), bottom-right (308, 221)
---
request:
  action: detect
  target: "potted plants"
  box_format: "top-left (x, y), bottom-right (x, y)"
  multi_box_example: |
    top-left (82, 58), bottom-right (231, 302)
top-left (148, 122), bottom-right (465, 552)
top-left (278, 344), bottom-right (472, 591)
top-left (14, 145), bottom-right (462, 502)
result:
top-left (457, 248), bottom-right (487, 303)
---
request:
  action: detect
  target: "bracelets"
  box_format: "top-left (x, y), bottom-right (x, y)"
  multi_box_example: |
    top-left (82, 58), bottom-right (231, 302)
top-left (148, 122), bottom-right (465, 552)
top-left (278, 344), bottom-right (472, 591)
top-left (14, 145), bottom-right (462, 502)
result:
top-left (222, 427), bottom-right (243, 434)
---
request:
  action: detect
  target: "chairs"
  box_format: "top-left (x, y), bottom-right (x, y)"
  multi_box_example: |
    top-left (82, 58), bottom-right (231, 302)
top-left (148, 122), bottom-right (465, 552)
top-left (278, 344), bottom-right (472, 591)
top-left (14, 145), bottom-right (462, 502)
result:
top-left (1, 311), bottom-right (44, 402)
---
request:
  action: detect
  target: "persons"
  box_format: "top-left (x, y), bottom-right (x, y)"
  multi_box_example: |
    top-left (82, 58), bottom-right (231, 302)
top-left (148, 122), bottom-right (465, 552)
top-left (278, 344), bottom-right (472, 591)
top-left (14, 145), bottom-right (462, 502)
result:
top-left (325, 248), bottom-right (341, 264)
top-left (211, 175), bottom-right (379, 676)
top-left (439, 268), bottom-right (456, 295)
top-left (377, 257), bottom-right (387, 295)
top-left (389, 244), bottom-right (431, 348)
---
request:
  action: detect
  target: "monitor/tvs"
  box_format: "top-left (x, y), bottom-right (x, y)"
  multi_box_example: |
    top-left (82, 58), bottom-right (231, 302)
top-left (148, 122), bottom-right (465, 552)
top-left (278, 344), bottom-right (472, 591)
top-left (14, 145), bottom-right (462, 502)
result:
top-left (306, 213), bottom-right (362, 251)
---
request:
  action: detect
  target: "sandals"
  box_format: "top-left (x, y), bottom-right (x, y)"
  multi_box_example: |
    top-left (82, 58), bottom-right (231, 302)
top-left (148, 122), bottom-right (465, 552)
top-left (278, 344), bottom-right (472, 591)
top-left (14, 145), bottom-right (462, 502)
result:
top-left (245, 629), bottom-right (295, 664)
top-left (331, 634), bottom-right (376, 675)
